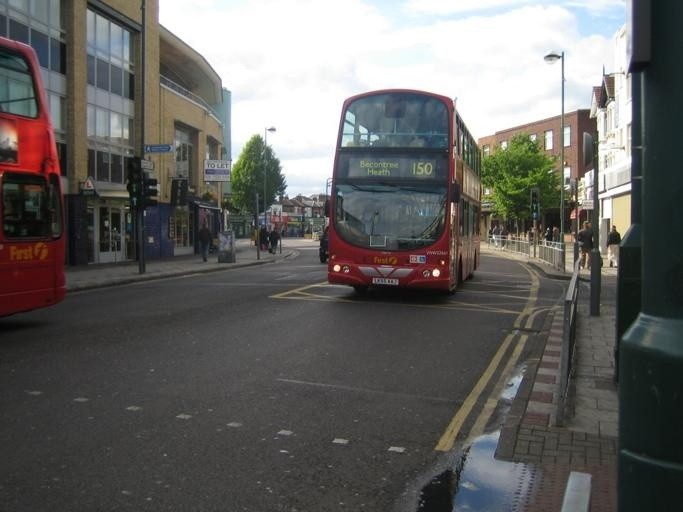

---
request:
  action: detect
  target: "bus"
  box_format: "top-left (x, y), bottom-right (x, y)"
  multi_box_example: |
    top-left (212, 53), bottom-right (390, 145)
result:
top-left (0, 36), bottom-right (69, 319)
top-left (327, 89), bottom-right (482, 294)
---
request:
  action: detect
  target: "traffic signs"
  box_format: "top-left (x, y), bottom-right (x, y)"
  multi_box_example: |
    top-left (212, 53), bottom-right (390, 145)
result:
top-left (140, 158), bottom-right (154, 170)
top-left (145, 144), bottom-right (171, 152)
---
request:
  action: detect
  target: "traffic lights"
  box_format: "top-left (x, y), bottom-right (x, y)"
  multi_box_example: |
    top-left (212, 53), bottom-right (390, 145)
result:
top-left (143, 176), bottom-right (159, 210)
top-left (126, 156), bottom-right (141, 208)
top-left (531, 189), bottom-right (539, 220)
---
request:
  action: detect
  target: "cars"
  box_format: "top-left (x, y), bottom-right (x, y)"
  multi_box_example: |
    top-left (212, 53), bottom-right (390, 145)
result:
top-left (319, 227), bottom-right (329, 264)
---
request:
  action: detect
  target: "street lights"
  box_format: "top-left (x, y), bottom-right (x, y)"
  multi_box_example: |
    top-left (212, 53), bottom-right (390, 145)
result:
top-left (569, 176), bottom-right (580, 267)
top-left (543, 49), bottom-right (565, 271)
top-left (264, 127), bottom-right (277, 225)
top-left (580, 129), bottom-right (601, 317)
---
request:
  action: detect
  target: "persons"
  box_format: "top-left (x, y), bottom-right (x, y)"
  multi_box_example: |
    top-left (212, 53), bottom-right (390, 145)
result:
top-left (195, 222), bottom-right (213, 263)
top-left (604, 224), bottom-right (620, 268)
top-left (486, 224), bottom-right (508, 252)
top-left (576, 219), bottom-right (592, 271)
top-left (536, 224), bottom-right (559, 246)
top-left (258, 224), bottom-right (280, 255)
top-left (345, 129), bottom-right (365, 147)
